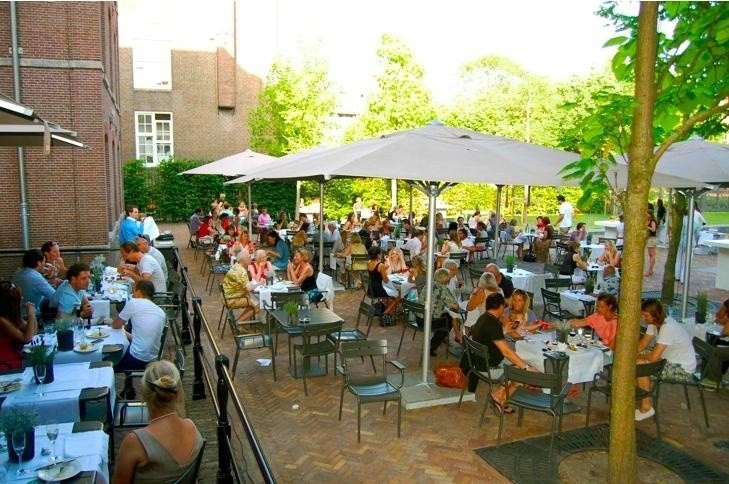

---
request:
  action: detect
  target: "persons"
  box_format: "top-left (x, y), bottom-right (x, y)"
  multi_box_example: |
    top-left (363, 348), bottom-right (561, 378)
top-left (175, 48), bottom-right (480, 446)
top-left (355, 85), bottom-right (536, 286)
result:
top-left (1, 203), bottom-right (204, 483)
top-left (188, 192), bottom-right (323, 336)
top-left (326, 194), bottom-right (728, 421)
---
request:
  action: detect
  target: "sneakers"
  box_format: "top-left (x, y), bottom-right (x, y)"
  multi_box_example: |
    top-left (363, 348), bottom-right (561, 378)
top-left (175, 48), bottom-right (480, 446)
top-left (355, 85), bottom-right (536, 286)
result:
top-left (235, 321), bottom-right (249, 333)
top-left (635, 407), bottom-right (655, 421)
top-left (120, 386), bottom-right (136, 400)
top-left (567, 389), bottom-right (580, 398)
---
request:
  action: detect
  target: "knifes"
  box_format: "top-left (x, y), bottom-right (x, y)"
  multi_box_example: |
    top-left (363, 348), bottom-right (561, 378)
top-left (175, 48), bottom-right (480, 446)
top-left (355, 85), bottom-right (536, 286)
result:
top-left (34, 458), bottom-right (75, 470)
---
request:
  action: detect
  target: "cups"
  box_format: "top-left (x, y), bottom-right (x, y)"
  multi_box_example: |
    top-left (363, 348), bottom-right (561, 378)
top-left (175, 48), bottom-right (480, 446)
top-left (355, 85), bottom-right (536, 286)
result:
top-left (108, 287), bottom-right (115, 293)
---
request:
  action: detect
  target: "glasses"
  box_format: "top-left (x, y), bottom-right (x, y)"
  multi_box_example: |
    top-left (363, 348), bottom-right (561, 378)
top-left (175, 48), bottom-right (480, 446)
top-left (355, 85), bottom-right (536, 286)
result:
top-left (138, 234), bottom-right (147, 240)
top-left (10, 283), bottom-right (16, 291)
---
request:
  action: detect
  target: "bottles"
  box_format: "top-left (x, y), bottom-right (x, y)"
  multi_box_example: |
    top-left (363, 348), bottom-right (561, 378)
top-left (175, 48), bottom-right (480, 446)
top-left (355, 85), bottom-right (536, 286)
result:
top-left (73, 305), bottom-right (84, 342)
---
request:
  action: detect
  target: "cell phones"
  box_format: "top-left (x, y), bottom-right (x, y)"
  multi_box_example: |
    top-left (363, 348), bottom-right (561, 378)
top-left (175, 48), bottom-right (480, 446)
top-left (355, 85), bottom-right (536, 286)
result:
top-left (511, 321), bottom-right (520, 329)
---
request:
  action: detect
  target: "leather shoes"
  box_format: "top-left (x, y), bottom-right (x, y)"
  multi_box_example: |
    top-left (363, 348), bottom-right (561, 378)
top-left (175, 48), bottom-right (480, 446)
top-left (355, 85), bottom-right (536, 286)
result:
top-left (430, 350), bottom-right (437, 357)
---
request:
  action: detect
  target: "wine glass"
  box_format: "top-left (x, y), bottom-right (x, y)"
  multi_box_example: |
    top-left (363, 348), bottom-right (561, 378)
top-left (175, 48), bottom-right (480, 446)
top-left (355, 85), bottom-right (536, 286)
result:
top-left (568, 327), bottom-right (578, 344)
top-left (551, 334), bottom-right (559, 351)
top-left (11, 428), bottom-right (27, 476)
top-left (35, 364), bottom-right (47, 397)
top-left (46, 420), bottom-right (59, 461)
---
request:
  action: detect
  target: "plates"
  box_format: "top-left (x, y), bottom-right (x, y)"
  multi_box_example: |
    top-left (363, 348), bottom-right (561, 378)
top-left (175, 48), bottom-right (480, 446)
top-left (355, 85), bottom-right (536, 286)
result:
top-left (73, 343), bottom-right (98, 353)
top-left (37, 459), bottom-right (81, 481)
top-left (87, 333), bottom-right (110, 338)
top-left (271, 285), bottom-right (284, 290)
top-left (0, 381), bottom-right (22, 397)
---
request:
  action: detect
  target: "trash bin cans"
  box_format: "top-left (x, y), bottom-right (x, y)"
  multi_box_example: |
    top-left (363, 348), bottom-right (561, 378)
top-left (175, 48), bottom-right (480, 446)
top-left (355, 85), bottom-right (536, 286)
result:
top-left (156, 232), bottom-right (174, 268)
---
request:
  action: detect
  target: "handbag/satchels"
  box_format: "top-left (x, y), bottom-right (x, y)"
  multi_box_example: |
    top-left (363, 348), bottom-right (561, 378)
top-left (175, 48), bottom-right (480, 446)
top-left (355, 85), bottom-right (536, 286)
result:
top-left (382, 315), bottom-right (398, 327)
top-left (434, 363), bottom-right (465, 389)
top-left (374, 302), bottom-right (384, 316)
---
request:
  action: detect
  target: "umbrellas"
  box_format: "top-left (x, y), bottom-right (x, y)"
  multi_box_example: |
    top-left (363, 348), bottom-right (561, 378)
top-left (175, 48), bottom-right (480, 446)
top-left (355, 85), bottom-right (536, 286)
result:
top-left (246, 117), bottom-right (717, 409)
top-left (222, 143), bottom-right (350, 272)
top-left (176, 148), bottom-right (278, 242)
top-left (605, 134), bottom-right (729, 317)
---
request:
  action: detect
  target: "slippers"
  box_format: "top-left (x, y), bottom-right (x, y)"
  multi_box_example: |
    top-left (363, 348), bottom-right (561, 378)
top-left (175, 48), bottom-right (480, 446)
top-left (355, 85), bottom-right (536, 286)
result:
top-left (489, 393), bottom-right (508, 409)
top-left (492, 400), bottom-right (515, 413)
top-left (455, 336), bottom-right (462, 344)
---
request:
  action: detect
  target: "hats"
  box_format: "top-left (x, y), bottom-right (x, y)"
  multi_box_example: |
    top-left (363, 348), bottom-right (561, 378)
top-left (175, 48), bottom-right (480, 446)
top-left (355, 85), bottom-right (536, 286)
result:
top-left (268, 231), bottom-right (276, 236)
